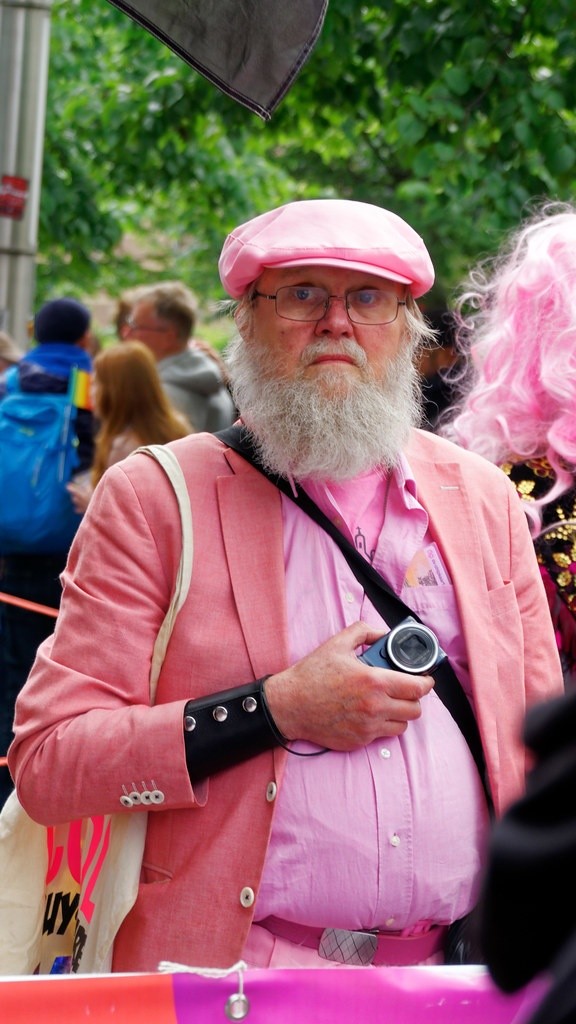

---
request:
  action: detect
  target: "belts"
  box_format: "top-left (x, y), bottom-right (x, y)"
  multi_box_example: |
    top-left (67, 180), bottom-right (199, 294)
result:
top-left (256, 911), bottom-right (446, 966)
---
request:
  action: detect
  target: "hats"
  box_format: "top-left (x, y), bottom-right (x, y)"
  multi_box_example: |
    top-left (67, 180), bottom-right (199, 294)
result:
top-left (218, 199), bottom-right (435, 300)
top-left (34, 296), bottom-right (90, 346)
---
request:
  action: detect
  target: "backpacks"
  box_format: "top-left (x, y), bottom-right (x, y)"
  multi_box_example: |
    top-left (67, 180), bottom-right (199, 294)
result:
top-left (0, 359), bottom-right (82, 554)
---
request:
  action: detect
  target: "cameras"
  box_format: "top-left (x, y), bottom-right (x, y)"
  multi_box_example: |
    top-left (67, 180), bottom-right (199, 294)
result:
top-left (356, 615), bottom-right (449, 677)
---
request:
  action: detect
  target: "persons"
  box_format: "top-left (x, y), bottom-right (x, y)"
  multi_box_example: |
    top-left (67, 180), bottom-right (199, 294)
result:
top-left (407, 277), bottom-right (473, 436)
top-left (432, 197), bottom-right (576, 710)
top-left (0, 279), bottom-right (241, 807)
top-left (4, 192), bottom-right (567, 980)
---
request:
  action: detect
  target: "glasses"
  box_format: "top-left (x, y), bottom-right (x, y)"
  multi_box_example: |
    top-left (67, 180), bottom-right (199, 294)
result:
top-left (125, 314), bottom-right (174, 334)
top-left (254, 284), bottom-right (407, 326)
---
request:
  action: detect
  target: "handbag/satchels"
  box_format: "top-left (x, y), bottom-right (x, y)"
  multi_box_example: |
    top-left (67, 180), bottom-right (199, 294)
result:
top-left (0, 447), bottom-right (195, 974)
